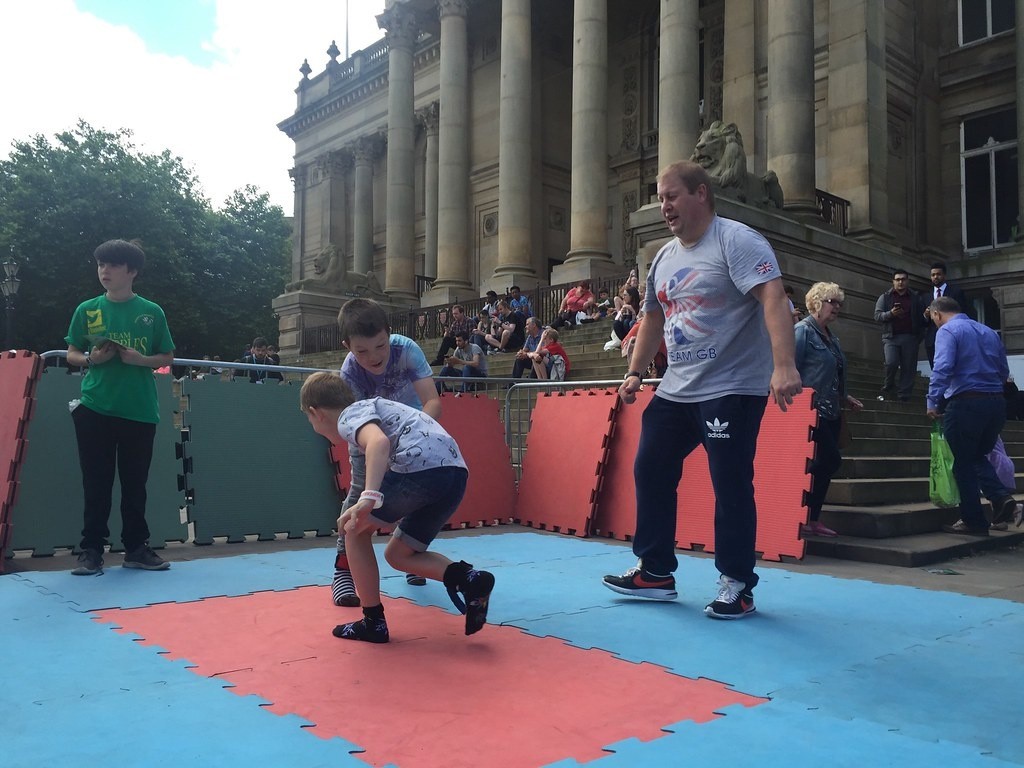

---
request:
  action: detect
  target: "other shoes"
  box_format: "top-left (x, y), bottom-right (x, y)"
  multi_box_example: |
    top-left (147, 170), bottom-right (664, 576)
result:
top-left (565, 320), bottom-right (572, 331)
top-left (881, 389), bottom-right (896, 399)
top-left (900, 392), bottom-right (911, 400)
top-left (429, 359), bottom-right (444, 366)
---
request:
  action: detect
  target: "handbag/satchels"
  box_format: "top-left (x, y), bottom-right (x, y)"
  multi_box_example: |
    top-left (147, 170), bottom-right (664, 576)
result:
top-left (929, 418), bottom-right (960, 508)
top-left (839, 411), bottom-right (853, 450)
top-left (1004, 381), bottom-right (1019, 398)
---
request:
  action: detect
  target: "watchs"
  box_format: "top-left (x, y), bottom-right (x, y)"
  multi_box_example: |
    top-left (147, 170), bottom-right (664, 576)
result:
top-left (624, 371), bottom-right (643, 383)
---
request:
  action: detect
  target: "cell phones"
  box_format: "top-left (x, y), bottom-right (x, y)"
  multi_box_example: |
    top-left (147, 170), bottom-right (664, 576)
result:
top-left (894, 303), bottom-right (900, 310)
top-left (444, 355), bottom-right (449, 358)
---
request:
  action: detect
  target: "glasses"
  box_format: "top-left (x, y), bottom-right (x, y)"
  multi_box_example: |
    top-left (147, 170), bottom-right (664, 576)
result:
top-left (822, 299), bottom-right (843, 306)
top-left (894, 278), bottom-right (906, 281)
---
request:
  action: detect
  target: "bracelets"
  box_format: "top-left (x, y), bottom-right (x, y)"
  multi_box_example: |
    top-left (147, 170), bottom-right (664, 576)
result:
top-left (632, 313), bottom-right (635, 315)
top-left (499, 321), bottom-right (503, 326)
top-left (86, 356), bottom-right (96, 368)
top-left (358, 490), bottom-right (384, 509)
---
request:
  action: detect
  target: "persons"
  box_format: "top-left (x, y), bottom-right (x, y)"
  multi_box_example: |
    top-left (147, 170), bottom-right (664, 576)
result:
top-left (925, 297), bottom-right (1023, 536)
top-left (430, 268), bottom-right (668, 391)
top-left (601, 159), bottom-right (803, 621)
top-left (64, 239), bottom-right (176, 576)
top-left (919, 263), bottom-right (969, 371)
top-left (331, 296), bottom-right (442, 607)
top-left (300, 372), bottom-right (495, 643)
top-left (784, 281), bottom-right (865, 538)
top-left (874, 268), bottom-right (925, 402)
top-left (188, 336), bottom-right (284, 385)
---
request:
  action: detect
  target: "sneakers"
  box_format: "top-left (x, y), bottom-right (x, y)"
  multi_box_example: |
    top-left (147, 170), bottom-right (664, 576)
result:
top-left (603, 564), bottom-right (678, 600)
top-left (71, 548), bottom-right (105, 577)
top-left (1013, 504), bottom-right (1023, 528)
top-left (703, 574), bottom-right (757, 619)
top-left (942, 518), bottom-right (989, 535)
top-left (124, 543), bottom-right (171, 571)
top-left (990, 521), bottom-right (1008, 531)
top-left (802, 521), bottom-right (837, 537)
top-left (990, 495), bottom-right (1016, 522)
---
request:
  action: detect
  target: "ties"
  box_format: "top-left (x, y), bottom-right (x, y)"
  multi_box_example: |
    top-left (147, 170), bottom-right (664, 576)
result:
top-left (937, 288), bottom-right (941, 297)
